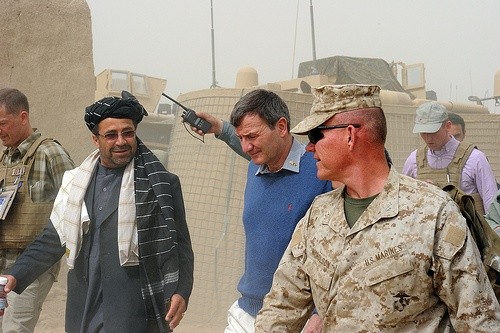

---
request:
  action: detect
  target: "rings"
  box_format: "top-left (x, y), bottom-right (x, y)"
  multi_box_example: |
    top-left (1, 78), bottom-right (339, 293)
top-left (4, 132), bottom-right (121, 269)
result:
top-left (182, 312), bottom-right (184, 316)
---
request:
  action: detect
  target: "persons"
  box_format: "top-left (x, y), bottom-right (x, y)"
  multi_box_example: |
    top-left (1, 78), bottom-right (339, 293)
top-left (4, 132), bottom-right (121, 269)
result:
top-left (253, 83), bottom-right (500, 333)
top-left (224, 89), bottom-right (333, 333)
top-left (484, 191), bottom-right (500, 237)
top-left (182, 111), bottom-right (393, 188)
top-left (402, 100), bottom-right (498, 214)
top-left (0, 96), bottom-right (195, 333)
top-left (0, 88), bottom-right (77, 333)
top-left (448, 112), bottom-right (465, 141)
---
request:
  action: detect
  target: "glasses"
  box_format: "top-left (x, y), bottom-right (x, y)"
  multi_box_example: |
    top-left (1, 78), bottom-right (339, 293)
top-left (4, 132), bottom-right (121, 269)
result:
top-left (96, 131), bottom-right (136, 139)
top-left (308, 124), bottom-right (361, 144)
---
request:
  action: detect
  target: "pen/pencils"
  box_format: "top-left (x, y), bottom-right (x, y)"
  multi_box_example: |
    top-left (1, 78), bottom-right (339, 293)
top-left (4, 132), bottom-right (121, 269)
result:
top-left (446, 167), bottom-right (449, 182)
top-left (14, 177), bottom-right (19, 184)
top-left (18, 182), bottom-right (23, 189)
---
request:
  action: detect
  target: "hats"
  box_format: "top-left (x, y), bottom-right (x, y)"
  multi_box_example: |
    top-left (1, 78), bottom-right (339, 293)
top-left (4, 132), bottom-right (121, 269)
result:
top-left (413, 103), bottom-right (448, 134)
top-left (84, 90), bottom-right (148, 132)
top-left (290, 83), bottom-right (382, 135)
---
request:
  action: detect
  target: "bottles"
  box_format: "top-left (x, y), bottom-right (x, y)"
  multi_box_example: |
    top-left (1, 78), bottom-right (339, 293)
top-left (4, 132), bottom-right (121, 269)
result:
top-left (0, 277), bottom-right (8, 316)
